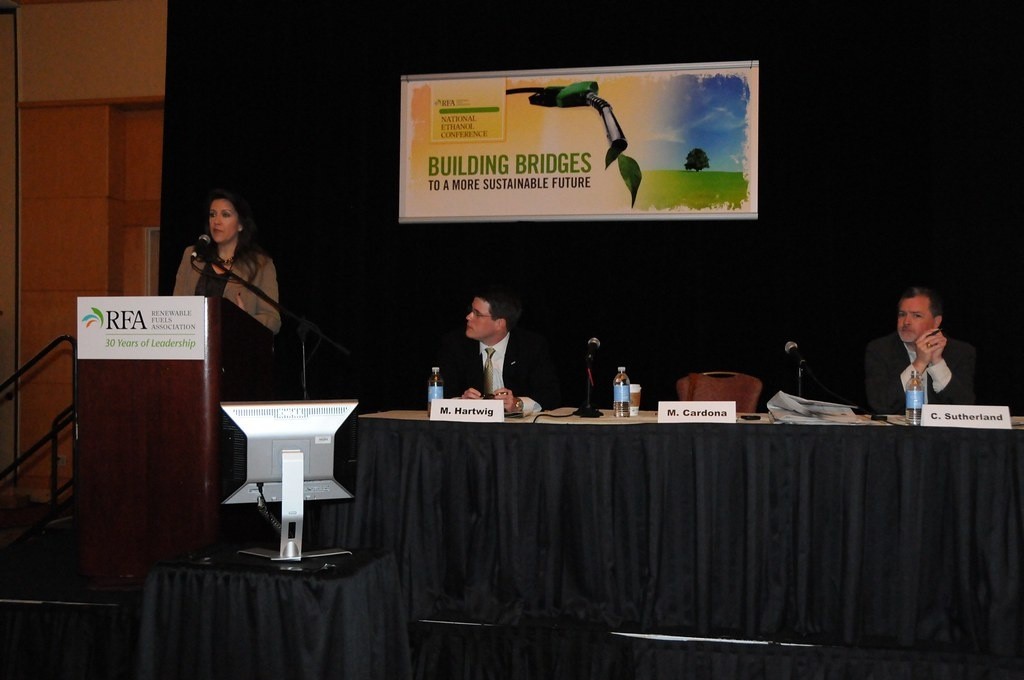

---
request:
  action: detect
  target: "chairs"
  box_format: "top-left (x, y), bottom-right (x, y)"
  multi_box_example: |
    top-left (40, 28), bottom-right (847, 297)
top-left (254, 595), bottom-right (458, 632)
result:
top-left (677, 372), bottom-right (762, 413)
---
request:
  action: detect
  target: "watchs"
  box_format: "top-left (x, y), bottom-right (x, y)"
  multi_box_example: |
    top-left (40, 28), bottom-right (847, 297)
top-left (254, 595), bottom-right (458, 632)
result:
top-left (515, 398), bottom-right (523, 411)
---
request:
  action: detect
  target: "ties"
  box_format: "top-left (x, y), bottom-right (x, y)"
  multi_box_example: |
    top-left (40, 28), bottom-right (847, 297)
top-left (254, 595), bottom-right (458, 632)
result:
top-left (484, 348), bottom-right (497, 398)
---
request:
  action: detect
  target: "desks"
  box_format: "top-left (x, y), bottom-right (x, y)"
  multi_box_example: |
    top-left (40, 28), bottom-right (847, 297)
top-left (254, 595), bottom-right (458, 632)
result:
top-left (134, 545), bottom-right (413, 680)
top-left (307, 411), bottom-right (1024, 645)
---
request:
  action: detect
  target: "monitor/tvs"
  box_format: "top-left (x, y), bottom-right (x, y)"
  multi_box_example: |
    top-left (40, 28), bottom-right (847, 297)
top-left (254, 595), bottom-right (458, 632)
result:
top-left (220, 399), bottom-right (353, 561)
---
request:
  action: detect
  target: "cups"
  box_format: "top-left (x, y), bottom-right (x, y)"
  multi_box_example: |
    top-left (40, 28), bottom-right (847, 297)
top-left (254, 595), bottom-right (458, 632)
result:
top-left (629, 384), bottom-right (642, 417)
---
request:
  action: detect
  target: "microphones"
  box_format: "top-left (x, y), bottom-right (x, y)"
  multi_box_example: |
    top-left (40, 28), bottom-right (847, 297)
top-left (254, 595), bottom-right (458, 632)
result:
top-left (785, 342), bottom-right (807, 366)
top-left (587, 338), bottom-right (600, 362)
top-left (190, 235), bottom-right (211, 262)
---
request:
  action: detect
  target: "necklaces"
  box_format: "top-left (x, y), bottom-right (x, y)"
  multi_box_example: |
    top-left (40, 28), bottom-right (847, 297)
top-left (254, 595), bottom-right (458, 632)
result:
top-left (216, 256), bottom-right (233, 264)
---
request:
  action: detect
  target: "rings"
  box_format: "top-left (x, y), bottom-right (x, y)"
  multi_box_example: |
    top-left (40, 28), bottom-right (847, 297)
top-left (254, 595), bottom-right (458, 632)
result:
top-left (927, 342), bottom-right (931, 348)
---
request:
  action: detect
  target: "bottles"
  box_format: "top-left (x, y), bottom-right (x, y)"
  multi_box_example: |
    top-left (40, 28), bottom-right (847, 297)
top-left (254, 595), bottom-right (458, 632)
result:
top-left (427, 367), bottom-right (444, 416)
top-left (613, 366), bottom-right (631, 417)
top-left (905, 370), bottom-right (925, 426)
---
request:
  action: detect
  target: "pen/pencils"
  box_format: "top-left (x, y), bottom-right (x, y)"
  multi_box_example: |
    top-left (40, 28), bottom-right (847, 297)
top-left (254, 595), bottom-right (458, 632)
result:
top-left (496, 392), bottom-right (508, 395)
top-left (928, 328), bottom-right (943, 336)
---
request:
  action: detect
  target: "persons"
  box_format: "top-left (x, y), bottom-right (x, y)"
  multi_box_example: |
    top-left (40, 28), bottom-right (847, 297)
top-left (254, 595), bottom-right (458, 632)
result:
top-left (438, 285), bottom-right (558, 410)
top-left (170, 189), bottom-right (282, 400)
top-left (864, 286), bottom-right (977, 414)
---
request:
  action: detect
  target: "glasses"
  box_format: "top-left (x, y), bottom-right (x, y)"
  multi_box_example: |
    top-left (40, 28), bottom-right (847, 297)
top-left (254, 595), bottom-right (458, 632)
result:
top-left (473, 311), bottom-right (495, 319)
